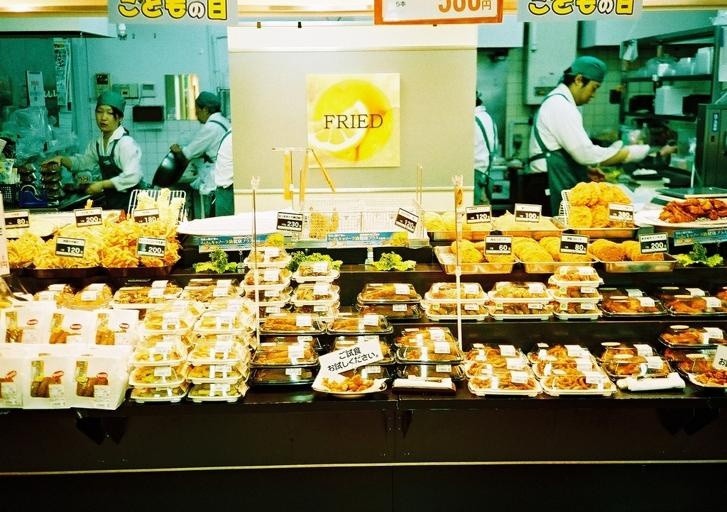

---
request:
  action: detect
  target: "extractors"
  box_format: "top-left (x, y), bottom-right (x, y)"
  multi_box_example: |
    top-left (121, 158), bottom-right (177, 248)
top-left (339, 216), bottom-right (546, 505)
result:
top-left (1, 16), bottom-right (110, 38)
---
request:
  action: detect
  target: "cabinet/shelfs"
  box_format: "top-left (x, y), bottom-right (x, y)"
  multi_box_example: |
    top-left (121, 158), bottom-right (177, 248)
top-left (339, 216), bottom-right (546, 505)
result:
top-left (0, 241), bottom-right (726, 509)
top-left (618, 30), bottom-right (715, 176)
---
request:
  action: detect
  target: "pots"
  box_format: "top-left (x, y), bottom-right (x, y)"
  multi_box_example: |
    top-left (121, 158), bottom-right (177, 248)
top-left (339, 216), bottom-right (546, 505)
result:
top-left (151, 146), bottom-right (189, 188)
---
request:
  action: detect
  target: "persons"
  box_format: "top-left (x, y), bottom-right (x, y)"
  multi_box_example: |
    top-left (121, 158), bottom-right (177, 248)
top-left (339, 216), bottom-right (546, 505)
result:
top-left (528, 55), bottom-right (651, 217)
top-left (213, 128), bottom-right (234, 216)
top-left (474, 91), bottom-right (499, 206)
top-left (170, 91), bottom-right (231, 216)
top-left (38, 90), bottom-right (145, 215)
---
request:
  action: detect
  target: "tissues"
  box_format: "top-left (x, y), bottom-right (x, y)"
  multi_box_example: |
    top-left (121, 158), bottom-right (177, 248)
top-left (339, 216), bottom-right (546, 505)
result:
top-left (133, 105), bottom-right (164, 130)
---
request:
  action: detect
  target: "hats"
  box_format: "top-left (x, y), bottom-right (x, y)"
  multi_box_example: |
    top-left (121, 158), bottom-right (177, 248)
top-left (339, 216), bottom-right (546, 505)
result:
top-left (196, 91), bottom-right (221, 110)
top-left (96, 91), bottom-right (126, 114)
top-left (567, 56), bottom-right (606, 82)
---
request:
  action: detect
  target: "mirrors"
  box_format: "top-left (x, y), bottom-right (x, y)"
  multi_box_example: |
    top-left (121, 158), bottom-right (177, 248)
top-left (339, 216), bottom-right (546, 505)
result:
top-left (165, 74), bottom-right (200, 121)
